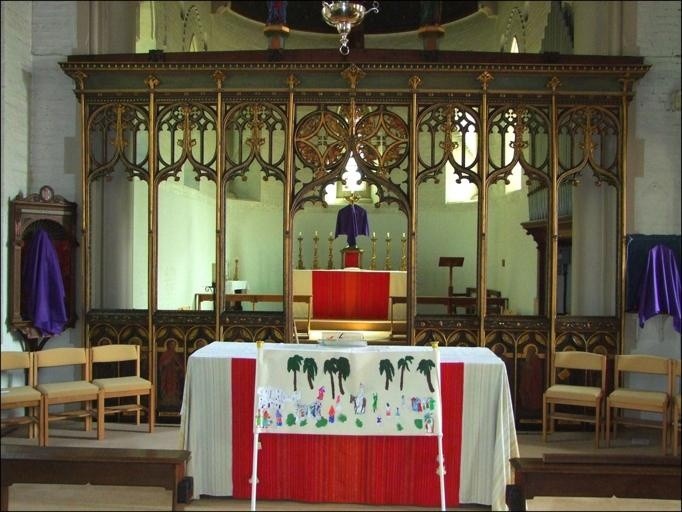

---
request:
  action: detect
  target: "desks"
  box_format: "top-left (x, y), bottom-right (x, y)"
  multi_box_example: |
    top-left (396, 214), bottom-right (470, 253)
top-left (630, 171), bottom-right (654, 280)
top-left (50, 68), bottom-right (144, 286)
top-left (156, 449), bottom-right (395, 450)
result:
top-left (183, 340), bottom-right (506, 503)
top-left (0, 444), bottom-right (191, 510)
top-left (503, 457), bottom-right (681, 512)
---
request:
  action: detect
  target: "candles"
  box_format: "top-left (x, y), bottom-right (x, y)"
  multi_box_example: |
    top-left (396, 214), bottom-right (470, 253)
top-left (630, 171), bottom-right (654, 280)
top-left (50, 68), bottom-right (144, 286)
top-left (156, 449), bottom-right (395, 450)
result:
top-left (373, 231), bottom-right (376, 238)
top-left (299, 232), bottom-right (302, 237)
top-left (387, 232), bottom-right (389, 237)
top-left (315, 231), bottom-right (318, 237)
top-left (403, 232), bottom-right (406, 238)
top-left (330, 232), bottom-right (333, 238)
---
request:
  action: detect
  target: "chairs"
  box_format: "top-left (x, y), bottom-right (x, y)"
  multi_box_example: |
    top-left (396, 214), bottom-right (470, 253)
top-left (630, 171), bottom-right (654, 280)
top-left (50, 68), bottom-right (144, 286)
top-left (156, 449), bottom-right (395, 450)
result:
top-left (542, 351), bottom-right (680, 458)
top-left (0, 343), bottom-right (155, 448)
